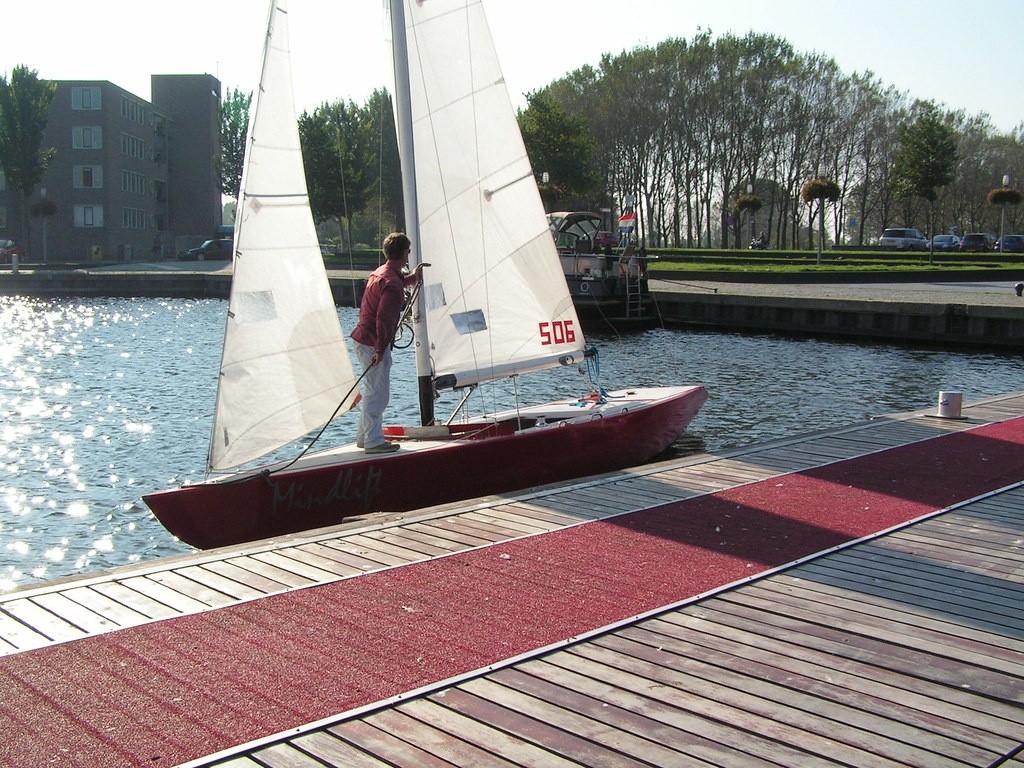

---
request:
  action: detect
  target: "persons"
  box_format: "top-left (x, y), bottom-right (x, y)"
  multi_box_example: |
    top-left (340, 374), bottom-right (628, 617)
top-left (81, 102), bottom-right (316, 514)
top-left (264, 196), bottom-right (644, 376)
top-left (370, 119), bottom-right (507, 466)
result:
top-left (757, 232), bottom-right (765, 249)
top-left (751, 238), bottom-right (755, 249)
top-left (350, 232), bottom-right (422, 454)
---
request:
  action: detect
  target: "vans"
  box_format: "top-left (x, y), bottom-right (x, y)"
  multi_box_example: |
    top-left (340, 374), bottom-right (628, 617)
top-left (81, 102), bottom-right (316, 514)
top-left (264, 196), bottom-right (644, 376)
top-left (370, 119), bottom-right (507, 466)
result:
top-left (958, 233), bottom-right (997, 254)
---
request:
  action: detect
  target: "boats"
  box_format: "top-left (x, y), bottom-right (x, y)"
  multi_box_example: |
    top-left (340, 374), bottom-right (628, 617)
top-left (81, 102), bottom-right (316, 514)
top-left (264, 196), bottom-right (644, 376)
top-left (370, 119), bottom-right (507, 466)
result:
top-left (547, 210), bottom-right (656, 325)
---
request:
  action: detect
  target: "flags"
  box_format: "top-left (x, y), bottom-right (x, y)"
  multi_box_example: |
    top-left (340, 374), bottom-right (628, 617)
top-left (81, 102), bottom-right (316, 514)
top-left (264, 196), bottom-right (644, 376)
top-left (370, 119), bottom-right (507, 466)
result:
top-left (618, 213), bottom-right (636, 233)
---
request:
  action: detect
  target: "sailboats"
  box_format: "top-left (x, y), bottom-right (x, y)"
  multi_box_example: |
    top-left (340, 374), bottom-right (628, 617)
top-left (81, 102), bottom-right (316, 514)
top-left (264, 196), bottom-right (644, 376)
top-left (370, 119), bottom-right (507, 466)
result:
top-left (141, 0), bottom-right (709, 549)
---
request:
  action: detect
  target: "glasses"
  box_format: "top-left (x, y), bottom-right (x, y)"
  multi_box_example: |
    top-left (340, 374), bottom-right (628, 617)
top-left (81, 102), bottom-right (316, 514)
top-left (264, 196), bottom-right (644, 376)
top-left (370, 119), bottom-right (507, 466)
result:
top-left (407, 249), bottom-right (411, 253)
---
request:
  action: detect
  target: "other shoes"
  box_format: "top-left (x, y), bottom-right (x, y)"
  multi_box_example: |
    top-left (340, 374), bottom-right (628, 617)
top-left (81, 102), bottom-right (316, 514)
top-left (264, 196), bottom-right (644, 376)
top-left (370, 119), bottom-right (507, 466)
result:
top-left (357, 440), bottom-right (391, 448)
top-left (365, 442), bottom-right (400, 454)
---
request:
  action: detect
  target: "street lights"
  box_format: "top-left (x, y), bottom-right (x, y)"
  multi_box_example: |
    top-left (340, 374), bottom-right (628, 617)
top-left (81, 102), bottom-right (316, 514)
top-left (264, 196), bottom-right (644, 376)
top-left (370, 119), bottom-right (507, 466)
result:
top-left (747, 182), bottom-right (757, 238)
top-left (41, 187), bottom-right (48, 263)
top-left (542, 171), bottom-right (549, 209)
top-left (1000, 175), bottom-right (1011, 237)
top-left (816, 163), bottom-right (826, 266)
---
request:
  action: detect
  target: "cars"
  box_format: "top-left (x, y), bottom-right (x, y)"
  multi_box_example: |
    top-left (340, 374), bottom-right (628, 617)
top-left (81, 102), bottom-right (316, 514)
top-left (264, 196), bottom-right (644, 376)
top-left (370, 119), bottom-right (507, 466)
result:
top-left (924, 236), bottom-right (963, 253)
top-left (0, 237), bottom-right (25, 265)
top-left (993, 235), bottom-right (1024, 253)
top-left (178, 239), bottom-right (234, 263)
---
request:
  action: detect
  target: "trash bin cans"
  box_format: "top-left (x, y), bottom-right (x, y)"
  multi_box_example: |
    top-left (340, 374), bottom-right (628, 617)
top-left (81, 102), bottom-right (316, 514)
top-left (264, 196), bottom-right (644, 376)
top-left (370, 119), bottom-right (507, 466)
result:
top-left (118, 245), bottom-right (132, 261)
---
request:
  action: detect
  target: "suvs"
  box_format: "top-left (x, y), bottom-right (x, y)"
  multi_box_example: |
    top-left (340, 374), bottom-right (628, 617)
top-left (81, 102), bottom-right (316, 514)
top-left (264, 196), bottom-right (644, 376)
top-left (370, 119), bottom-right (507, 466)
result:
top-left (879, 227), bottom-right (927, 254)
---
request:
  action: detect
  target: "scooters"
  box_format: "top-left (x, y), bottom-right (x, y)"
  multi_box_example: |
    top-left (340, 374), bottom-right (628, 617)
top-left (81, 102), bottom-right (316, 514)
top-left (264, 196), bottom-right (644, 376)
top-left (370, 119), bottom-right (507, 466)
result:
top-left (747, 238), bottom-right (769, 250)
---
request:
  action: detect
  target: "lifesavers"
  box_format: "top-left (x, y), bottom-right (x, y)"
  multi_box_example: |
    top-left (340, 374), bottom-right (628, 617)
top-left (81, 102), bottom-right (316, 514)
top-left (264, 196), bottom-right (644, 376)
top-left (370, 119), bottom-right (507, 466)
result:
top-left (383, 425), bottom-right (450, 440)
